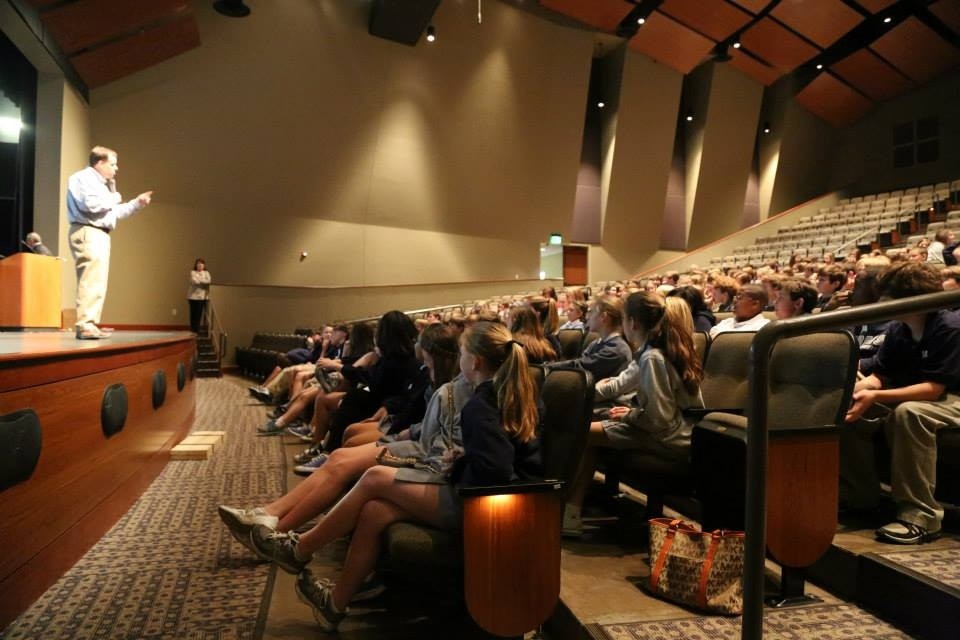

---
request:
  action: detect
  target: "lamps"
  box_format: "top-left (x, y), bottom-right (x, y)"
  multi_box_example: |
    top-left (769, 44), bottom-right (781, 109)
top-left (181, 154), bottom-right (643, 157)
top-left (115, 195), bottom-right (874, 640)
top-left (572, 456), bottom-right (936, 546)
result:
top-left (426, 24), bottom-right (435, 43)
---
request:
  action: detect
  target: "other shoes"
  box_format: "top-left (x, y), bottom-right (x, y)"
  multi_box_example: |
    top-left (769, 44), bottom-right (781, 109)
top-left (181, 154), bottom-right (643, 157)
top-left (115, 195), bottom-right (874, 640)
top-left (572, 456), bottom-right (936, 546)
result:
top-left (76, 325), bottom-right (112, 339)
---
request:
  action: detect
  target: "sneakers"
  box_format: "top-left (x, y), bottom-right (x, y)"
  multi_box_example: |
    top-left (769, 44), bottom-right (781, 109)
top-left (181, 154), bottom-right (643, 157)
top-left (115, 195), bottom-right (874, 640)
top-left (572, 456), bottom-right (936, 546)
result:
top-left (250, 524), bottom-right (314, 575)
top-left (295, 570), bottom-right (349, 633)
top-left (218, 386), bottom-right (328, 474)
top-left (218, 505), bottom-right (279, 534)
top-left (876, 520), bottom-right (942, 543)
top-left (562, 505), bottom-right (584, 536)
top-left (347, 570), bottom-right (388, 604)
top-left (231, 530), bottom-right (271, 562)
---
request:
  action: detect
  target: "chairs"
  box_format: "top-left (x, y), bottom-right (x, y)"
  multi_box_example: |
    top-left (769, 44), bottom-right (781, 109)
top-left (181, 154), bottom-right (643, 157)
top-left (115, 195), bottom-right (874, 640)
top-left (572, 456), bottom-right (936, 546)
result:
top-left (231, 178), bottom-right (960, 638)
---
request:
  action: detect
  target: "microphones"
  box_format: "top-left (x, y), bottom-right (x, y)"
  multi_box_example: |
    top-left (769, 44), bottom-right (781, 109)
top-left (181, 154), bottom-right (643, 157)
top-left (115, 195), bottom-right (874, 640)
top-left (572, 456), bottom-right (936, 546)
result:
top-left (20, 239), bottom-right (38, 253)
top-left (108, 179), bottom-right (116, 193)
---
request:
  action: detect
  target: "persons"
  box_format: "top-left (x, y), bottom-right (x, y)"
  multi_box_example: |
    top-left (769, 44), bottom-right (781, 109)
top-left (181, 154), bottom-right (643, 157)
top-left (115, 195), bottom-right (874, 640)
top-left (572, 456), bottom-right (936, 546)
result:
top-left (346, 365), bottom-right (483, 605)
top-left (845, 264), bottom-right (960, 543)
top-left (66, 145), bottom-right (154, 341)
top-left (26, 232), bottom-right (53, 257)
top-left (245, 225), bottom-right (960, 477)
top-left (559, 290), bottom-right (707, 540)
top-left (185, 258), bottom-right (212, 336)
top-left (216, 357), bottom-right (435, 554)
top-left (250, 318), bottom-right (552, 632)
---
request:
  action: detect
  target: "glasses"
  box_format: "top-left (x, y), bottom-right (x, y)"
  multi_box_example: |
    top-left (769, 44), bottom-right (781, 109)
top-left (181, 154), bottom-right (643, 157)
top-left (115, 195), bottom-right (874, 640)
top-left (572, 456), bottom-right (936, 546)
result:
top-left (733, 295), bottom-right (758, 303)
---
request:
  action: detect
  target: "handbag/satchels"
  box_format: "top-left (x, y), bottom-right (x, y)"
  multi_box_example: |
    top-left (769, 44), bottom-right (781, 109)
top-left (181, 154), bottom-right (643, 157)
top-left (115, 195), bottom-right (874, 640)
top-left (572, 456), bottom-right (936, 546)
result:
top-left (641, 518), bottom-right (745, 617)
